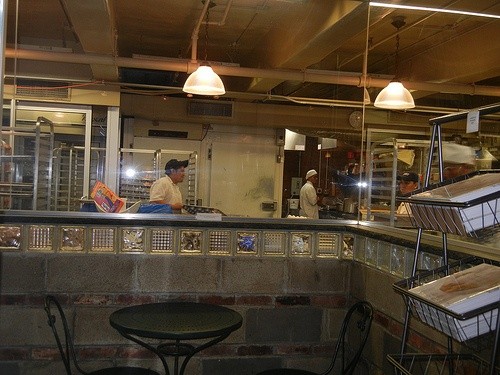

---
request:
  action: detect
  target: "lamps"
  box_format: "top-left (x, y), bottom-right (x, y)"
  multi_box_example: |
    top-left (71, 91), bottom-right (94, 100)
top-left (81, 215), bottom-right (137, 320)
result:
top-left (182, 0), bottom-right (226, 95)
top-left (374, 21), bottom-right (416, 110)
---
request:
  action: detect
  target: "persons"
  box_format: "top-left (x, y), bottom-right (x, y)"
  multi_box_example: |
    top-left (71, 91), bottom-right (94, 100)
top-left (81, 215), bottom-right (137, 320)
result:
top-left (300, 169), bottom-right (323, 218)
top-left (150, 159), bottom-right (189, 212)
top-left (396, 172), bottom-right (419, 215)
top-left (443, 167), bottom-right (451, 180)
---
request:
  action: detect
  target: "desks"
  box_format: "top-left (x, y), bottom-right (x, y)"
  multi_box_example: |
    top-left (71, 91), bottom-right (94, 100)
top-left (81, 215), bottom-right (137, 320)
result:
top-left (107, 300), bottom-right (244, 375)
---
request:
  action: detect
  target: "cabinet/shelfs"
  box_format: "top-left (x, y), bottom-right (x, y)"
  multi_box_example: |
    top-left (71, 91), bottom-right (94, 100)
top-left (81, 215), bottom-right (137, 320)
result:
top-left (386, 103), bottom-right (500, 375)
top-left (366, 138), bottom-right (469, 226)
top-left (0, 116), bottom-right (79, 209)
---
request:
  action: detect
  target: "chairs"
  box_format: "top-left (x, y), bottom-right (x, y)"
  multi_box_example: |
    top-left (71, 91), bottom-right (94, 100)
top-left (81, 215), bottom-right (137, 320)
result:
top-left (258, 300), bottom-right (373, 375)
top-left (44, 294), bottom-right (161, 375)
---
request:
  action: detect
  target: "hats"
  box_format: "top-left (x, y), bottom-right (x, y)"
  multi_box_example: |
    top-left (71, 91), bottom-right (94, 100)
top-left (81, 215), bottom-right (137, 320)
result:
top-left (165, 159), bottom-right (189, 175)
top-left (396, 171), bottom-right (419, 182)
top-left (305, 169), bottom-right (318, 180)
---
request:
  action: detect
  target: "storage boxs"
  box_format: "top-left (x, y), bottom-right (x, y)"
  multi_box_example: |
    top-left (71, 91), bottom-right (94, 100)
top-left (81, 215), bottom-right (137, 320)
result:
top-left (411, 263), bottom-right (500, 342)
top-left (409, 173), bottom-right (500, 233)
